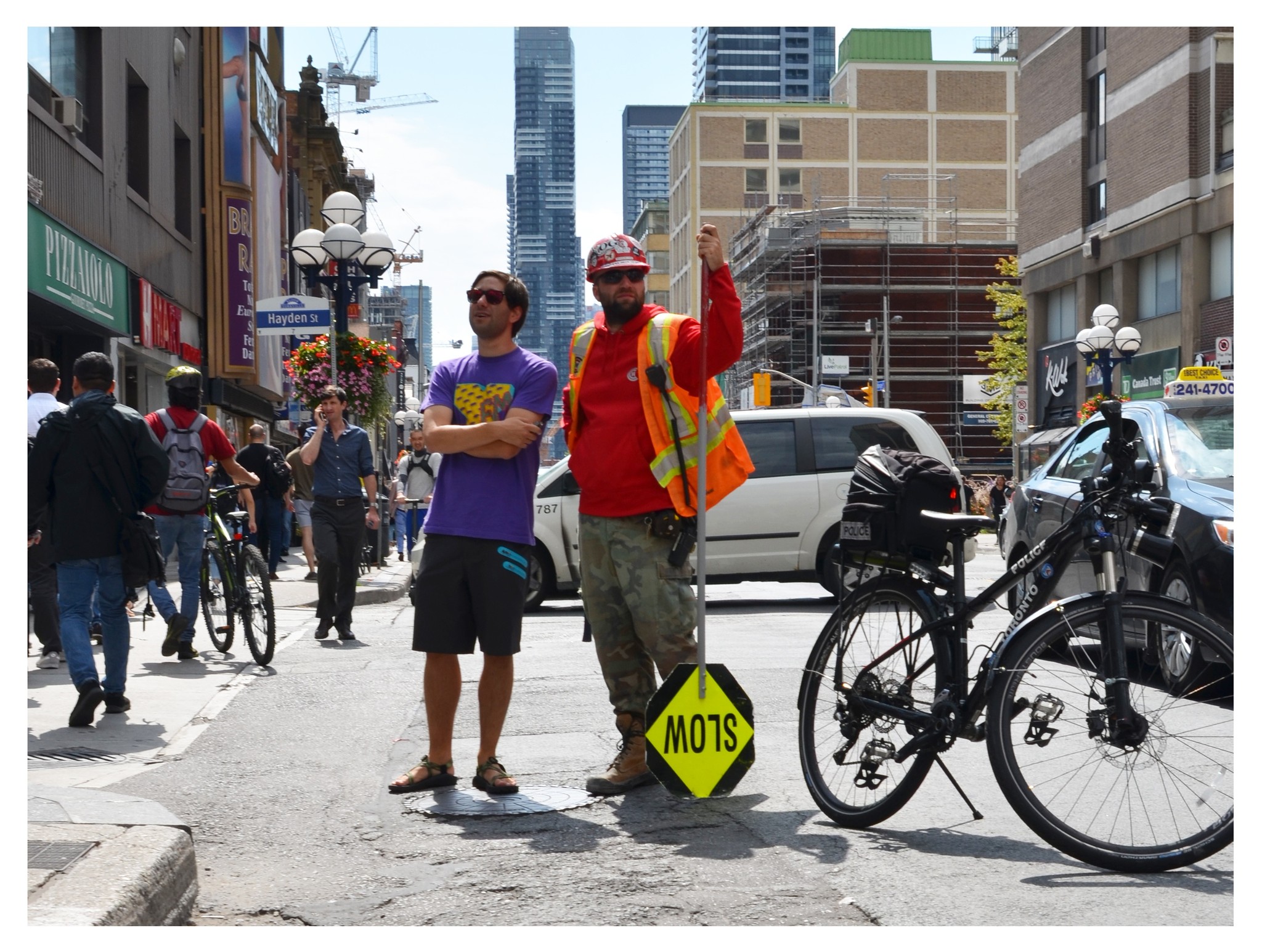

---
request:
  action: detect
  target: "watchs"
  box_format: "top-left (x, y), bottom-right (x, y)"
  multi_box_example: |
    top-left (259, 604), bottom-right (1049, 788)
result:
top-left (371, 503), bottom-right (379, 510)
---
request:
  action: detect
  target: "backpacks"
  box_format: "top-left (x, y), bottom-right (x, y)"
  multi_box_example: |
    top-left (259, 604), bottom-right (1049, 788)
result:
top-left (156, 408), bottom-right (208, 514)
top-left (262, 445), bottom-right (290, 496)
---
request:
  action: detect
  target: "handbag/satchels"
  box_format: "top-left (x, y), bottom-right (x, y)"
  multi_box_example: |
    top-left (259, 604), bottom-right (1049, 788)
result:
top-left (123, 510), bottom-right (168, 589)
top-left (839, 444), bottom-right (981, 571)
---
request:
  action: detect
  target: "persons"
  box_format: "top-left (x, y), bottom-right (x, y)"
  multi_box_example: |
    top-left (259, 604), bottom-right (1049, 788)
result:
top-left (387, 270), bottom-right (559, 794)
top-left (563, 224), bottom-right (757, 797)
top-left (28, 351), bottom-right (171, 728)
top-left (298, 386), bottom-right (381, 639)
top-left (222, 48), bottom-right (250, 185)
top-left (27, 358), bottom-right (443, 667)
top-left (142, 366), bottom-right (261, 659)
top-left (989, 475), bottom-right (1011, 545)
top-left (960, 475), bottom-right (980, 516)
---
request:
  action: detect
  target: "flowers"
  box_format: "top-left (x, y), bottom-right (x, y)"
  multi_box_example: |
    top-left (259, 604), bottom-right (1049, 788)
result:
top-left (282, 332), bottom-right (402, 440)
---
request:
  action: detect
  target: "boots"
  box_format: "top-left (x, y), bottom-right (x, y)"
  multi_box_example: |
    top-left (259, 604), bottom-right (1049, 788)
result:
top-left (586, 712), bottom-right (654, 793)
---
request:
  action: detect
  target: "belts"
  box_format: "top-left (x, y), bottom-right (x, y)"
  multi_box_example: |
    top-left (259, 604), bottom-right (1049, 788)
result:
top-left (314, 495), bottom-right (363, 506)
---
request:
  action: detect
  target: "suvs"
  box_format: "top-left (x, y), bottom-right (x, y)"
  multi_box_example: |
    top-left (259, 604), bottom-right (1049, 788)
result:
top-left (410, 408), bottom-right (978, 614)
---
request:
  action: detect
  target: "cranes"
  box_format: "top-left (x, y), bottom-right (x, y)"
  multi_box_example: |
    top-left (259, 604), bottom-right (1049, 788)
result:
top-left (317, 27), bottom-right (440, 135)
top-left (366, 199), bottom-right (425, 321)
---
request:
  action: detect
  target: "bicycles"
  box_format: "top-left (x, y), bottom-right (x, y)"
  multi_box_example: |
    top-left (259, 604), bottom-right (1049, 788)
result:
top-left (359, 544), bottom-right (373, 575)
top-left (200, 482), bottom-right (276, 666)
top-left (796, 398), bottom-right (1234, 875)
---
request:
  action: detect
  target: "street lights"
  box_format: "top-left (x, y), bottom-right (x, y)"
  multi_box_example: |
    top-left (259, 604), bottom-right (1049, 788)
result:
top-left (1074, 303), bottom-right (1143, 401)
top-left (290, 190), bottom-right (395, 426)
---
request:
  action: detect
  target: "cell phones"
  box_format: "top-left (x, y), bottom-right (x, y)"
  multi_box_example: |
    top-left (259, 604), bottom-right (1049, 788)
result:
top-left (320, 413), bottom-right (324, 421)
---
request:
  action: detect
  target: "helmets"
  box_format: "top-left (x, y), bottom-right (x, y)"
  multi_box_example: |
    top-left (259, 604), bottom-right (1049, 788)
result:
top-left (165, 365), bottom-right (203, 389)
top-left (304, 426), bottom-right (316, 437)
top-left (586, 234), bottom-right (652, 282)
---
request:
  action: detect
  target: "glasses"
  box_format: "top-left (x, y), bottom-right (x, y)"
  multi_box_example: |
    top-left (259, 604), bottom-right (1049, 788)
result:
top-left (467, 289), bottom-right (507, 305)
top-left (599, 268), bottom-right (644, 283)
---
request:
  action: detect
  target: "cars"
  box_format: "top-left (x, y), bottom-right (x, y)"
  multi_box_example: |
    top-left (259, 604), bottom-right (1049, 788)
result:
top-left (997, 464), bottom-right (1043, 560)
top-left (1007, 366), bottom-right (1235, 702)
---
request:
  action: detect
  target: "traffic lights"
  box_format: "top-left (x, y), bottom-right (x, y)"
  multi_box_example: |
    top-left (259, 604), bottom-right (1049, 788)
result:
top-left (861, 381), bottom-right (873, 408)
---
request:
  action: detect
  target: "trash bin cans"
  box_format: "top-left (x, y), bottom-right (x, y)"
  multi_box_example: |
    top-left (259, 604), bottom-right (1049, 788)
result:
top-left (361, 488), bottom-right (391, 563)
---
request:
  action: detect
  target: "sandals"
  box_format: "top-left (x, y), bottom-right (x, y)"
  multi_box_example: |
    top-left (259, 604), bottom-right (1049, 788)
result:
top-left (388, 755), bottom-right (458, 792)
top-left (472, 756), bottom-right (518, 795)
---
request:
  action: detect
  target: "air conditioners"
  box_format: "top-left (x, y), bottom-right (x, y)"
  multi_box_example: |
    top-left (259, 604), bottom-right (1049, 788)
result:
top-left (52, 97), bottom-right (83, 133)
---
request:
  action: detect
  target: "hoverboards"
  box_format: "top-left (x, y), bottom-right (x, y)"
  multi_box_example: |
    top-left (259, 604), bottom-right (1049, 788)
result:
top-left (392, 497), bottom-right (423, 549)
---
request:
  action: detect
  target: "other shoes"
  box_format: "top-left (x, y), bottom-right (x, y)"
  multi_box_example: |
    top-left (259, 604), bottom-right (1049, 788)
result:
top-left (37, 651), bottom-right (65, 669)
top-left (68, 679), bottom-right (105, 727)
top-left (212, 585), bottom-right (219, 592)
top-left (315, 616), bottom-right (334, 638)
top-left (305, 572), bottom-right (318, 580)
top-left (279, 557), bottom-right (286, 563)
top-left (281, 552), bottom-right (289, 556)
top-left (338, 630), bottom-right (355, 640)
top-left (92, 623), bottom-right (102, 645)
top-left (399, 553), bottom-right (403, 561)
top-left (103, 692), bottom-right (130, 714)
top-left (162, 614), bottom-right (199, 659)
top-left (269, 571), bottom-right (278, 580)
